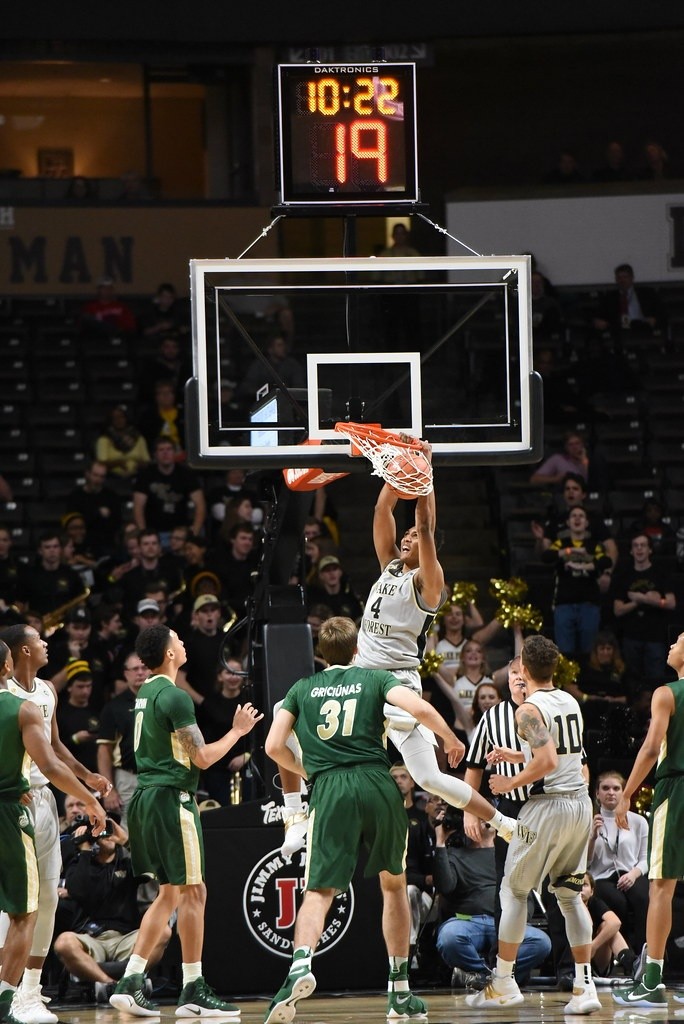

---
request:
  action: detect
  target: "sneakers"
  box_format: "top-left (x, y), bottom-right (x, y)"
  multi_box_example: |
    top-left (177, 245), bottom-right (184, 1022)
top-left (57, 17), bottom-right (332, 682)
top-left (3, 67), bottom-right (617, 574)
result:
top-left (465, 967), bottom-right (524, 1007)
top-left (280, 801), bottom-right (310, 855)
top-left (263, 949), bottom-right (316, 1024)
top-left (175, 1017), bottom-right (241, 1024)
top-left (496, 816), bottom-right (517, 845)
top-left (613, 1005), bottom-right (668, 1024)
top-left (8, 982), bottom-right (58, 1023)
top-left (110, 974), bottom-right (161, 1016)
top-left (611, 974), bottom-right (668, 1007)
top-left (673, 994), bottom-right (684, 1003)
top-left (564, 978), bottom-right (602, 1014)
top-left (387, 1016), bottom-right (428, 1024)
top-left (386, 962), bottom-right (428, 1017)
top-left (564, 1013), bottom-right (603, 1024)
top-left (175, 976), bottom-right (240, 1017)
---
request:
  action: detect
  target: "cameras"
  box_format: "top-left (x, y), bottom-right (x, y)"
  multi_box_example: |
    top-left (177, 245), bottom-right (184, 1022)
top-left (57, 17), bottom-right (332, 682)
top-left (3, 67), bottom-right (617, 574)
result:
top-left (68, 814), bottom-right (113, 844)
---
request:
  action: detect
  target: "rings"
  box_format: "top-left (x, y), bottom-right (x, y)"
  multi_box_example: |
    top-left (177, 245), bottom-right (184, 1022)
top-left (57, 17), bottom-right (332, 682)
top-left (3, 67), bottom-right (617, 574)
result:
top-left (110, 784), bottom-right (113, 788)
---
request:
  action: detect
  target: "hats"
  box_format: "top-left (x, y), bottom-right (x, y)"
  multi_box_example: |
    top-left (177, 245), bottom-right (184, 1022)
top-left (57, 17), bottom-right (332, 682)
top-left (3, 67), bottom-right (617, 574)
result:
top-left (320, 556), bottom-right (339, 571)
top-left (66, 657), bottom-right (90, 680)
top-left (61, 513), bottom-right (81, 530)
top-left (72, 608), bottom-right (90, 623)
top-left (138, 599), bottom-right (159, 614)
top-left (194, 594), bottom-right (219, 613)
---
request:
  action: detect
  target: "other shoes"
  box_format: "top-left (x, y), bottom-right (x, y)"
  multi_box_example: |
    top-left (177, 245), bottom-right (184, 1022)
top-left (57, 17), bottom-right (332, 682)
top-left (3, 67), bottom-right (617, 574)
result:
top-left (94, 975), bottom-right (154, 1003)
top-left (633, 943), bottom-right (647, 980)
top-left (451, 968), bottom-right (485, 986)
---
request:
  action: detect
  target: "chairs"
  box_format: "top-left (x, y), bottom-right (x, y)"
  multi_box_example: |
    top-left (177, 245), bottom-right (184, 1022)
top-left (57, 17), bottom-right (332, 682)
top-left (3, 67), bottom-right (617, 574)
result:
top-left (0, 291), bottom-right (230, 549)
top-left (453, 281), bottom-right (684, 573)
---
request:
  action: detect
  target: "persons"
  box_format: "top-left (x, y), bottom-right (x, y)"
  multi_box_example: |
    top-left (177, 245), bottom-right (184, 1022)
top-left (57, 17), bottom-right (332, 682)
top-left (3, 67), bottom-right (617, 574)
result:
top-left (0, 624), bottom-right (113, 1024)
top-left (611, 630), bottom-right (684, 1008)
top-left (109, 623), bottom-right (264, 1018)
top-left (263, 616), bottom-right (465, 1024)
top-left (466, 635), bottom-right (602, 1014)
top-left (0, 223), bottom-right (684, 1003)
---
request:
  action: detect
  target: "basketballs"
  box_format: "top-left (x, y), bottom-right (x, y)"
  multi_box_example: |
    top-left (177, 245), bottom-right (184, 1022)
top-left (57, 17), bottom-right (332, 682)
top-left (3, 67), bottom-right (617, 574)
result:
top-left (384, 449), bottom-right (433, 500)
